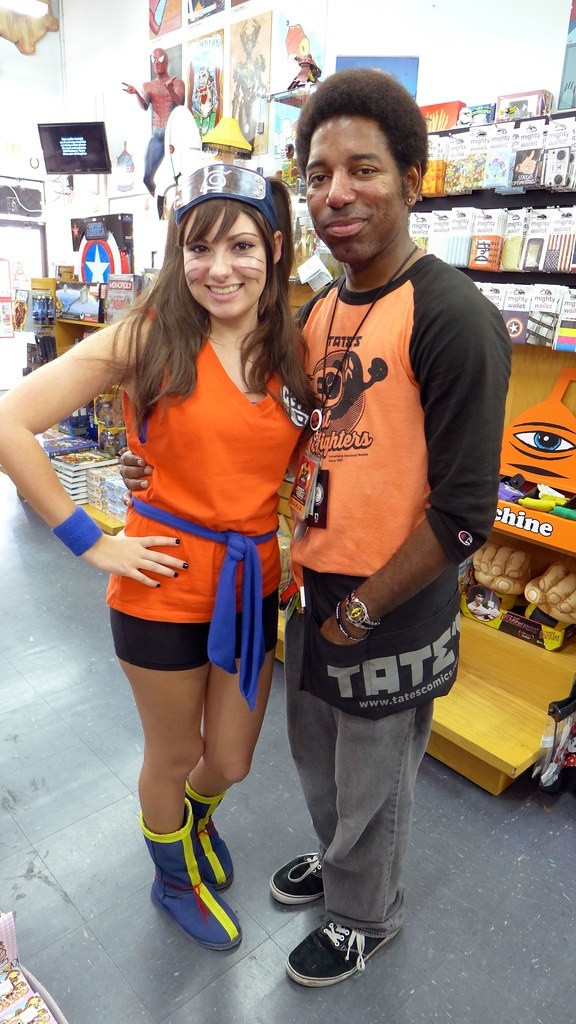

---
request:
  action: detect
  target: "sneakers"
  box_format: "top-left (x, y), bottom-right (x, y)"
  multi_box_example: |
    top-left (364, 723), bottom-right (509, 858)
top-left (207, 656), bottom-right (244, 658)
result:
top-left (287, 918), bottom-right (398, 988)
top-left (268, 851), bottom-right (323, 902)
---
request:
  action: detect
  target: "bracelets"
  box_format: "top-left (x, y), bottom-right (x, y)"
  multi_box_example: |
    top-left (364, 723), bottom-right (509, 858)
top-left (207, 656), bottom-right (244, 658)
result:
top-left (120, 70), bottom-right (509, 988)
top-left (52, 508), bottom-right (103, 557)
top-left (336, 602), bottom-right (370, 643)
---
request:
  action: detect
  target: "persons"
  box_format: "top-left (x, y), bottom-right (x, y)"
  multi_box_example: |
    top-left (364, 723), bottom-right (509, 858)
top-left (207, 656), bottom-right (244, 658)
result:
top-left (0, 165), bottom-right (319, 952)
top-left (467, 589), bottom-right (499, 622)
top-left (122, 48), bottom-right (186, 198)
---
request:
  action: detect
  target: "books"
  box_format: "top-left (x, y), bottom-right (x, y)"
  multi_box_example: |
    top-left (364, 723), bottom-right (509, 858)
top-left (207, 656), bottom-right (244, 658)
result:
top-left (34, 430), bottom-right (118, 507)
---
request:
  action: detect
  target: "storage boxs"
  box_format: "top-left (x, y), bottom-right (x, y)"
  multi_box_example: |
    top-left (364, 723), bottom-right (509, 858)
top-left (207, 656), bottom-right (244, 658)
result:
top-left (420, 89), bottom-right (552, 134)
top-left (52, 213), bottom-right (159, 457)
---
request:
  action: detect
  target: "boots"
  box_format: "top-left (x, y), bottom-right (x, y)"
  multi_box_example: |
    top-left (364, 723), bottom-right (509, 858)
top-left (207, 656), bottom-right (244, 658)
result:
top-left (141, 782), bottom-right (244, 952)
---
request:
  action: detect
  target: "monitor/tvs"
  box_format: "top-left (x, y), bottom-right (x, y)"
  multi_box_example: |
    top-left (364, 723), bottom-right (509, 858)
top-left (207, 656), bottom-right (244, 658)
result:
top-left (37, 121), bottom-right (112, 174)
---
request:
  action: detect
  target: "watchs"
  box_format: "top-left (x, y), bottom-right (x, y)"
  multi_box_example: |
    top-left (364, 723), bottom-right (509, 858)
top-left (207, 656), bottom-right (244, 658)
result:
top-left (345, 591), bottom-right (380, 630)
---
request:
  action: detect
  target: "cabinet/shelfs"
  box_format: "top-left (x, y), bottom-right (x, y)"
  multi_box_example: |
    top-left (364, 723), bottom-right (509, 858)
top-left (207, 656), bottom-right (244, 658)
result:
top-left (424, 497), bottom-right (576, 797)
top-left (4, 316), bottom-right (125, 538)
